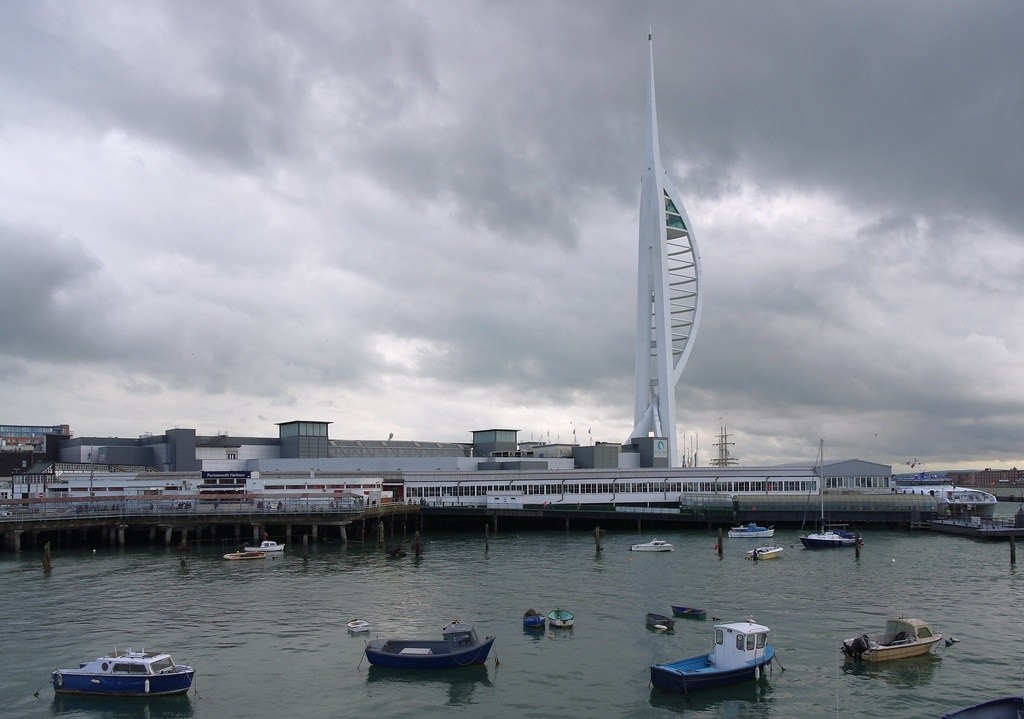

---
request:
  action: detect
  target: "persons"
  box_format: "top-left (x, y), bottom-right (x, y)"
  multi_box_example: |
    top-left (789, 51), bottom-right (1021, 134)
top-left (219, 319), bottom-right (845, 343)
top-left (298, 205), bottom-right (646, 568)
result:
top-left (277, 501), bottom-right (282, 512)
top-left (177, 501), bottom-right (192, 511)
top-left (420, 498), bottom-right (426, 506)
top-left (150, 503), bottom-right (153, 510)
top-left (946, 507), bottom-right (951, 519)
top-left (266, 502), bottom-right (272, 513)
top-left (408, 498), bottom-right (411, 505)
top-left (393, 497), bottom-right (396, 506)
top-left (76, 503), bottom-right (120, 516)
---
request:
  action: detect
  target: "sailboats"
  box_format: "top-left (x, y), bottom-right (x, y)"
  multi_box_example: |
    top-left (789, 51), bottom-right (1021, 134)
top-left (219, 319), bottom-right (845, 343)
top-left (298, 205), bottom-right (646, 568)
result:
top-left (797, 438), bottom-right (864, 550)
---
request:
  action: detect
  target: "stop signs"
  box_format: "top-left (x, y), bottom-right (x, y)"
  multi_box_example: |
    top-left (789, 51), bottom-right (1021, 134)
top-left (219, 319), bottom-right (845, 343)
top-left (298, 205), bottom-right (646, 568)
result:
top-left (39, 492), bottom-right (44, 498)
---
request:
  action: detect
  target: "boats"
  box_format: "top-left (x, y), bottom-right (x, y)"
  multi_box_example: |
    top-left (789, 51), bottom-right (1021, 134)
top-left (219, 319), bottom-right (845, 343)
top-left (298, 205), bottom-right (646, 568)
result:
top-left (650, 615), bottom-right (776, 694)
top-left (50, 645), bottom-right (200, 698)
top-left (222, 550), bottom-right (267, 560)
top-left (547, 607), bottom-right (575, 628)
top-left (347, 618), bottom-right (370, 633)
top-left (243, 533), bottom-right (286, 553)
top-left (727, 521), bottom-right (776, 539)
top-left (744, 544), bottom-right (784, 561)
top-left (840, 612), bottom-right (943, 662)
top-left (670, 603), bottom-right (708, 621)
top-left (630, 537), bottom-right (674, 553)
top-left (645, 612), bottom-right (674, 635)
top-left (363, 618), bottom-right (497, 668)
top-left (520, 608), bottom-right (546, 628)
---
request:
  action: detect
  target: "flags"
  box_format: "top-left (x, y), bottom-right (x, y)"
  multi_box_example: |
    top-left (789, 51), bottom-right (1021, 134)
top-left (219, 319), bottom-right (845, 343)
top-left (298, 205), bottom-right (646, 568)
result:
top-left (588, 428), bottom-right (591, 433)
top-left (573, 429), bottom-right (575, 434)
